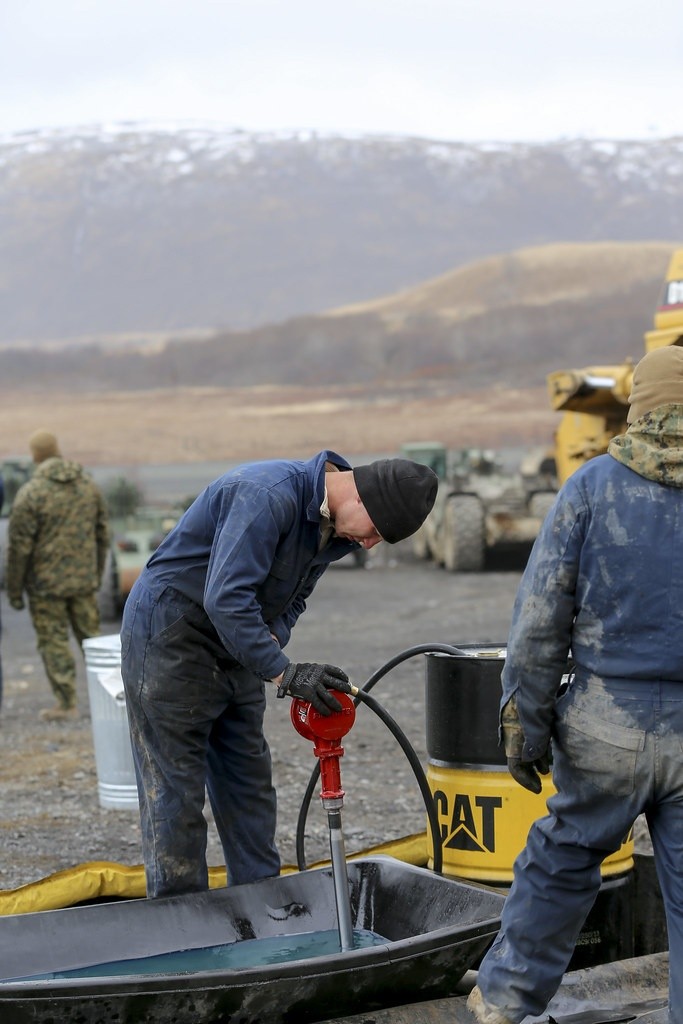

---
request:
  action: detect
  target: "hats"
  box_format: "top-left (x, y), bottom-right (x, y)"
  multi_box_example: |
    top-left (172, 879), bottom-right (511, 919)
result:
top-left (626, 346), bottom-right (683, 424)
top-left (31, 433), bottom-right (59, 457)
top-left (353, 458), bottom-right (438, 544)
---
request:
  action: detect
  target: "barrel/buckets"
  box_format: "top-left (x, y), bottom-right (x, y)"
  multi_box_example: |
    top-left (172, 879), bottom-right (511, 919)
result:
top-left (426, 643), bottom-right (641, 971)
top-left (81, 632), bottom-right (209, 809)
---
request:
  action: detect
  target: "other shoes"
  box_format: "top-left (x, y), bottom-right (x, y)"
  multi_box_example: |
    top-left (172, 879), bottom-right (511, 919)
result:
top-left (7, 593), bottom-right (24, 609)
top-left (467, 984), bottom-right (516, 1024)
top-left (39, 702), bottom-right (83, 723)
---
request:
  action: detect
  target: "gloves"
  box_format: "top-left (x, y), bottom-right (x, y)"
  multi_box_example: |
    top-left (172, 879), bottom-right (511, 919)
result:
top-left (277, 662), bottom-right (351, 717)
top-left (508, 755), bottom-right (555, 794)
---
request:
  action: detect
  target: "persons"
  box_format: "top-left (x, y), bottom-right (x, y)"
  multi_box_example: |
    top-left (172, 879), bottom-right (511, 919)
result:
top-left (465, 342), bottom-right (683, 1024)
top-left (7, 431), bottom-right (113, 720)
top-left (120, 449), bottom-right (438, 898)
